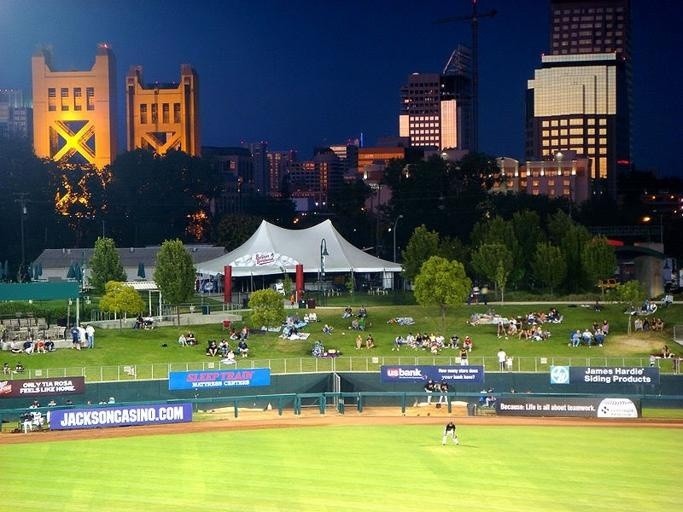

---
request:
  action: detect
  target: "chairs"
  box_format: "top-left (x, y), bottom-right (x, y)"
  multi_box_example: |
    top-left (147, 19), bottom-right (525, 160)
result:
top-left (1, 316), bottom-right (65, 341)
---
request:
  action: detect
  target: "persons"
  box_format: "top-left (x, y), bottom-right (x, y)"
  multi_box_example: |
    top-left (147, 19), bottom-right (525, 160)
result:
top-left (23, 412), bottom-right (33, 434)
top-left (132, 314), bottom-right (154, 330)
top-left (423, 378), bottom-right (434, 405)
top-left (33, 412), bottom-right (45, 432)
top-left (442, 421), bottom-right (460, 445)
top-left (476, 385), bottom-right (535, 408)
top-left (4, 320), bottom-right (117, 409)
top-left (438, 379), bottom-right (449, 405)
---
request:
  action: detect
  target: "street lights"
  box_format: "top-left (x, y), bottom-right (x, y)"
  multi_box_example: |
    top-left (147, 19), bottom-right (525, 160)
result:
top-left (321, 239), bottom-right (328, 293)
top-left (21, 201), bottom-right (27, 266)
top-left (393, 215), bottom-right (404, 289)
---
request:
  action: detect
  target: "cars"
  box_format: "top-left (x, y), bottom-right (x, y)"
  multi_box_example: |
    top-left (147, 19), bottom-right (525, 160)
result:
top-left (595, 278), bottom-right (620, 290)
